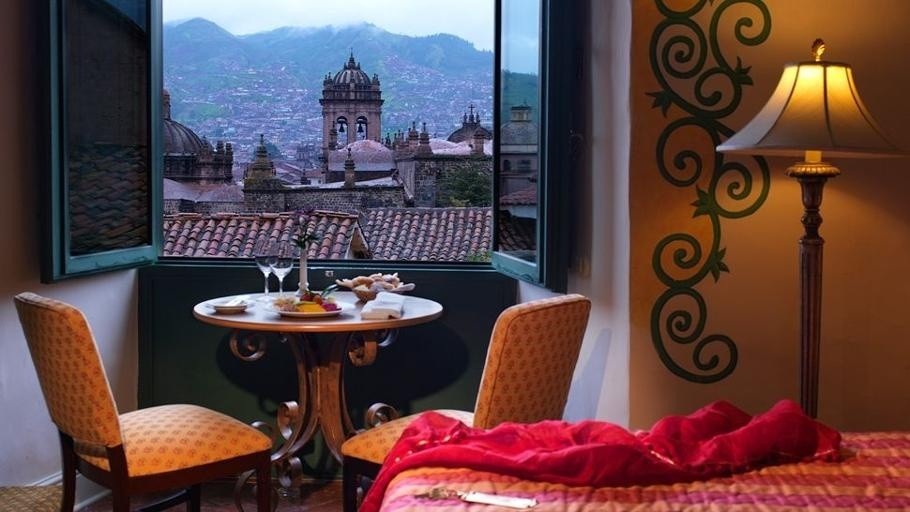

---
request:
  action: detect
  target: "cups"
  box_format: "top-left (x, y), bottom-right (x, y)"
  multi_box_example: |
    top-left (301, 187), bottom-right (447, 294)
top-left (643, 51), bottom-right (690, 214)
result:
top-left (267, 239), bottom-right (295, 301)
top-left (253, 238), bottom-right (279, 304)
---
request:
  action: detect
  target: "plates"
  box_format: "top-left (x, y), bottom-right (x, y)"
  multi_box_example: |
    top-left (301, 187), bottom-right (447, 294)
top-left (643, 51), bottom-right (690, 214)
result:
top-left (205, 300), bottom-right (255, 314)
top-left (276, 302), bottom-right (346, 317)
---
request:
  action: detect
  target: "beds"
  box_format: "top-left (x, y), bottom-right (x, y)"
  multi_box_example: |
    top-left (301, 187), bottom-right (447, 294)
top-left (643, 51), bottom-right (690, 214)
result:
top-left (354, 397), bottom-right (909, 512)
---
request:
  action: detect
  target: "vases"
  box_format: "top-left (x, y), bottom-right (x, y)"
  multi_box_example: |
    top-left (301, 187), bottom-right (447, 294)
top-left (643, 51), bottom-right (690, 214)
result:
top-left (295, 248), bottom-right (313, 297)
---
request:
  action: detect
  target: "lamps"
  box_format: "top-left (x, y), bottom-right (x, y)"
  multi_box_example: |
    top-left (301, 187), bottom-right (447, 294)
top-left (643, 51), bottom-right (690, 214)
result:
top-left (716, 37), bottom-right (906, 427)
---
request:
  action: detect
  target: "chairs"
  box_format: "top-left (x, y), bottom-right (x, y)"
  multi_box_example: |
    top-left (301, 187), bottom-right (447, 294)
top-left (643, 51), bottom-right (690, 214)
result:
top-left (16, 291), bottom-right (276, 512)
top-left (338, 294), bottom-right (591, 511)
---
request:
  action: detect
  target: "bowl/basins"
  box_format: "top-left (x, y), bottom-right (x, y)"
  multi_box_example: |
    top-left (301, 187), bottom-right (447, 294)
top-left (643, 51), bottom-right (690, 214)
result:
top-left (351, 282), bottom-right (415, 303)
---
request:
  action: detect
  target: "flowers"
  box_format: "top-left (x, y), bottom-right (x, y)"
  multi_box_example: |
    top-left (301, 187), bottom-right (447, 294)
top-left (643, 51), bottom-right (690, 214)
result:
top-left (289, 205), bottom-right (325, 248)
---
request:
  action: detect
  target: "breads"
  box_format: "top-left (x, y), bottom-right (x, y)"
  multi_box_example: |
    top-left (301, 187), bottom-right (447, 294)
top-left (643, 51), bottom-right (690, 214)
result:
top-left (369, 282), bottom-right (393, 290)
top-left (353, 276), bottom-right (372, 287)
top-left (370, 275), bottom-right (384, 283)
top-left (384, 275), bottom-right (400, 287)
